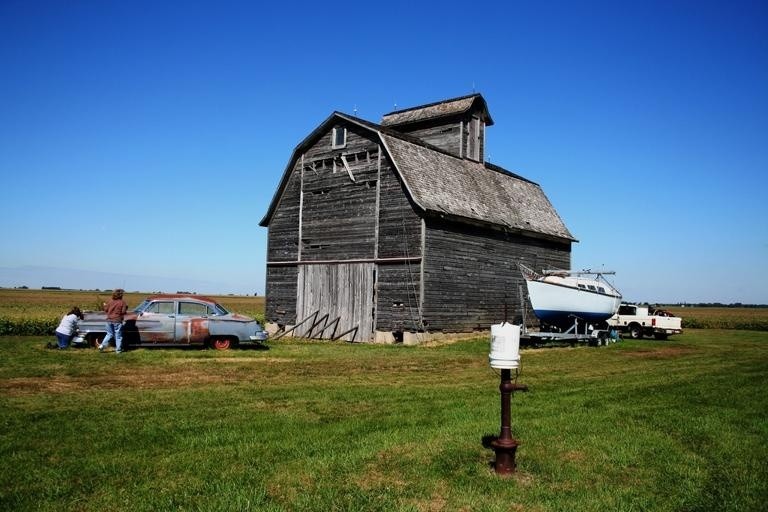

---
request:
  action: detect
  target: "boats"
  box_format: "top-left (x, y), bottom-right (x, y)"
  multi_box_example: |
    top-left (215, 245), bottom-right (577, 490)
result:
top-left (518, 263), bottom-right (623, 330)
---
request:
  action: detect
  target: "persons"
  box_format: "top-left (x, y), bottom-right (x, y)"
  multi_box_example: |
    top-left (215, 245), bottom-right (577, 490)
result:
top-left (47, 307), bottom-right (81, 350)
top-left (98, 289), bottom-right (128, 354)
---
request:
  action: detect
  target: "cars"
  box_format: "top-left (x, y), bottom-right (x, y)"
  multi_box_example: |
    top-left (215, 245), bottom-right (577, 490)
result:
top-left (71, 294), bottom-right (270, 351)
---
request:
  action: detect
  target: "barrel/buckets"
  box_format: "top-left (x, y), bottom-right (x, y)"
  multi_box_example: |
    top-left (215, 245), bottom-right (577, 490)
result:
top-left (488, 321), bottom-right (523, 381)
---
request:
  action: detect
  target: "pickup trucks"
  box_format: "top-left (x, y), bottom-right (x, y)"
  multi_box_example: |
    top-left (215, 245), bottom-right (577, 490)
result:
top-left (607, 303), bottom-right (682, 340)
